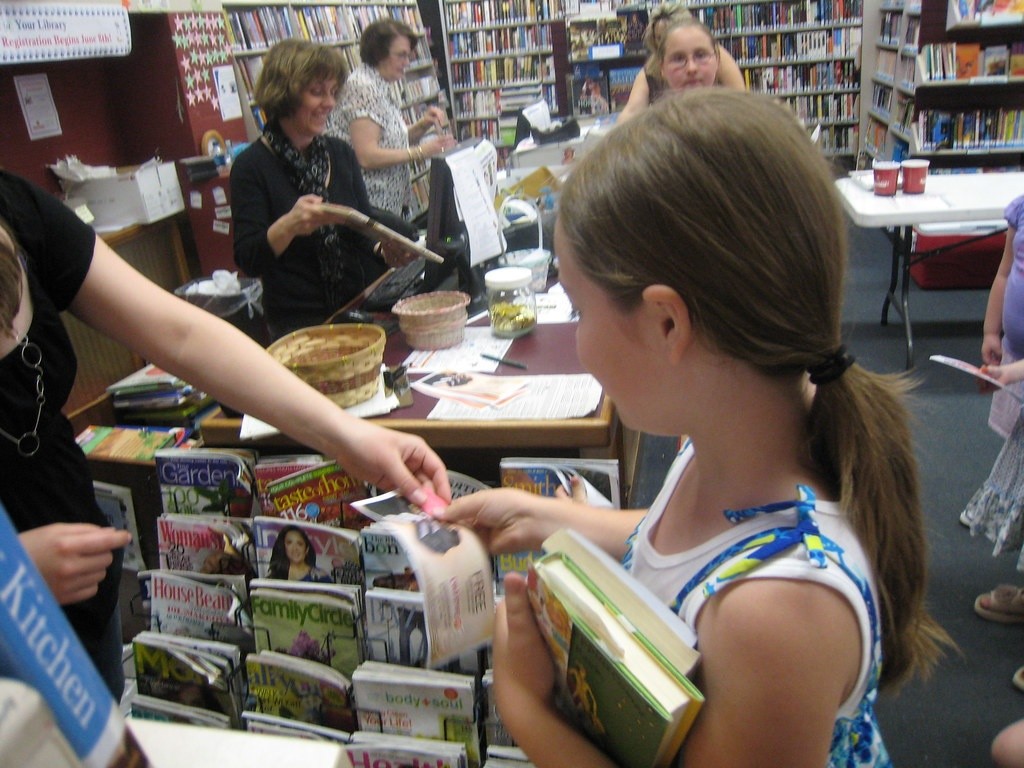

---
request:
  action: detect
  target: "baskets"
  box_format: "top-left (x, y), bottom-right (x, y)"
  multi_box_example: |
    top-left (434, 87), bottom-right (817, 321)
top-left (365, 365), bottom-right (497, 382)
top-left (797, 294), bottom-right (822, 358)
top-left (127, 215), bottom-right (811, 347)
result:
top-left (497, 193), bottom-right (551, 292)
top-left (266, 324), bottom-right (386, 409)
top-left (392, 291), bottom-right (471, 350)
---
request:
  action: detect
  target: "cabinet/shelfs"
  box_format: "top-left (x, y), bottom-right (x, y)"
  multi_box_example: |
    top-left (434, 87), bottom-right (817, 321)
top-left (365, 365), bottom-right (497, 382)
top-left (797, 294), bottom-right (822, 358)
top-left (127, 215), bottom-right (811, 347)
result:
top-left (213, 1), bottom-right (457, 235)
top-left (859, 1), bottom-right (1023, 250)
top-left (436, 0), bottom-right (865, 171)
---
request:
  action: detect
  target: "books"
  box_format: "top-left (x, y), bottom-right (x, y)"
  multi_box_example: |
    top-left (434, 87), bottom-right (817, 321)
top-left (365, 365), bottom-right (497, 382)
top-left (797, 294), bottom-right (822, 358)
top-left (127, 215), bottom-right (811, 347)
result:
top-left (443, 0), bottom-right (750, 174)
top-left (525, 528), bottom-right (706, 767)
top-left (228, 0), bottom-right (451, 215)
top-left (92, 446), bottom-right (621, 768)
top-left (76, 368), bottom-right (208, 466)
top-left (856, 0), bottom-right (1024, 175)
top-left (690, 0), bottom-right (862, 154)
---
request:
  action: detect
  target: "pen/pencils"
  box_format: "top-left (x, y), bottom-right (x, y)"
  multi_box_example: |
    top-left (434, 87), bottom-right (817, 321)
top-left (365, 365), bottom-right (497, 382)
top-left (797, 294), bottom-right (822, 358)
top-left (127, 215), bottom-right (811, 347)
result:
top-left (480, 352), bottom-right (528, 371)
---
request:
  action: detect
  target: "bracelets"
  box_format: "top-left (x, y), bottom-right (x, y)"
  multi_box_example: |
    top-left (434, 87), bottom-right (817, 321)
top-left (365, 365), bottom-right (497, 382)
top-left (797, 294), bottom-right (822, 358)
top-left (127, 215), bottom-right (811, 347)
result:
top-left (416, 145), bottom-right (425, 160)
top-left (413, 147), bottom-right (422, 162)
top-left (408, 149), bottom-right (413, 165)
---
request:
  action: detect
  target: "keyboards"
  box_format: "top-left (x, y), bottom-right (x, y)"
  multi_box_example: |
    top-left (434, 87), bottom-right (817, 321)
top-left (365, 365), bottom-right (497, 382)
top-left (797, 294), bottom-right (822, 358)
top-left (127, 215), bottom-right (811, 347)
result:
top-left (358, 254), bottom-right (426, 312)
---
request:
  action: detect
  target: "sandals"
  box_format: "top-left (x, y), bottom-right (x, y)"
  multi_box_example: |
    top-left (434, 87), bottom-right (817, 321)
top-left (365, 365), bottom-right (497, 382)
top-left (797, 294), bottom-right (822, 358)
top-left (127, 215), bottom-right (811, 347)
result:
top-left (974, 584), bottom-right (1024, 624)
top-left (1012, 666), bottom-right (1024, 693)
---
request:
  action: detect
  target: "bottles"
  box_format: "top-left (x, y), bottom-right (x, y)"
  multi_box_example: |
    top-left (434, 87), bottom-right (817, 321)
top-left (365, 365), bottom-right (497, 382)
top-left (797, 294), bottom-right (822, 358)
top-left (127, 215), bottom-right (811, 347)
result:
top-left (485, 267), bottom-right (538, 338)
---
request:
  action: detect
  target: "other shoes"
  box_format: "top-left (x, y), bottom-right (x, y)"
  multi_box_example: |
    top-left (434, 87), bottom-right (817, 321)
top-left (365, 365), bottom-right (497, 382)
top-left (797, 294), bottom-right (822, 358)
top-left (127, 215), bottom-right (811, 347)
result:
top-left (960, 509), bottom-right (977, 528)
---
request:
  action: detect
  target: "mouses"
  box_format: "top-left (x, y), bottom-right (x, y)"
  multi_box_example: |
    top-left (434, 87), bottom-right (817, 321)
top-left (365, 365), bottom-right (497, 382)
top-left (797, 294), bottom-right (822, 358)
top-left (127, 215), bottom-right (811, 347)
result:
top-left (331, 312), bottom-right (374, 324)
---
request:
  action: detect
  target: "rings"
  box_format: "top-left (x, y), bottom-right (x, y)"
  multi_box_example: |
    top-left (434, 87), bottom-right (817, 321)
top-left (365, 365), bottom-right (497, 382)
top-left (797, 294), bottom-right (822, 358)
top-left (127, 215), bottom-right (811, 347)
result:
top-left (442, 147), bottom-right (444, 153)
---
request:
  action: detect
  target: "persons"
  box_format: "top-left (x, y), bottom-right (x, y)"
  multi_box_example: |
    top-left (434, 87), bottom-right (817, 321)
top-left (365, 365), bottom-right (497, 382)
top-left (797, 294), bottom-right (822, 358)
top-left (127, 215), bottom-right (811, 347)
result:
top-left (268, 526), bottom-right (333, 584)
top-left (0, 169), bottom-right (450, 704)
top-left (959, 193), bottom-right (1024, 768)
top-left (331, 20), bottom-right (457, 215)
top-left (658, 18), bottom-right (721, 93)
top-left (229, 39), bottom-right (419, 336)
top-left (433, 88), bottom-right (949, 768)
top-left (619, 4), bottom-right (744, 121)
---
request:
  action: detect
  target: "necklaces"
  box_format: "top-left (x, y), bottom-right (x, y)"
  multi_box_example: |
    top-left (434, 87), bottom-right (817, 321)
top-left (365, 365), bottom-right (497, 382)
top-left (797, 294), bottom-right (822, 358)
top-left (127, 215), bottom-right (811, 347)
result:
top-left (0, 327), bottom-right (46, 456)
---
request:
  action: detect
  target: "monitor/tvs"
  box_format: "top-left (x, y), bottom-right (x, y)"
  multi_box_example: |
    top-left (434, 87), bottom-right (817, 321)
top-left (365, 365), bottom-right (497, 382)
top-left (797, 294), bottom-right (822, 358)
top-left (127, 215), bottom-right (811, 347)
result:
top-left (423, 135), bottom-right (500, 317)
top-left (514, 104), bottom-right (539, 151)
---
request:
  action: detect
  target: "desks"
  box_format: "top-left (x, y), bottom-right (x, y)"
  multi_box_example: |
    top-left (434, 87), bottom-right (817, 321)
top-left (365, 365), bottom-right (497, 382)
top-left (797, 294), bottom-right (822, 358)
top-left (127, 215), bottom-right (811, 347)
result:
top-left (199, 249), bottom-right (627, 510)
top-left (835, 171), bottom-right (1022, 373)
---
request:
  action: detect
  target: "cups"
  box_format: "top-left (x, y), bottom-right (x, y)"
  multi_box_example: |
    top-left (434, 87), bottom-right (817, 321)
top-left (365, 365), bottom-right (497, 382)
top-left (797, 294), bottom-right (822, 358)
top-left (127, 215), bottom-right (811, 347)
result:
top-left (900, 159), bottom-right (930, 195)
top-left (872, 161), bottom-right (900, 195)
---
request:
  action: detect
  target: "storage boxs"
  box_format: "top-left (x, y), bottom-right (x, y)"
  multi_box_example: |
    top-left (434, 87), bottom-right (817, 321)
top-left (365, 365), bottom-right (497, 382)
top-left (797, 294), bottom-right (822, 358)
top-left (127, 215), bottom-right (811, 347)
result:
top-left (56, 158), bottom-right (186, 224)
top-left (902, 219), bottom-right (1009, 291)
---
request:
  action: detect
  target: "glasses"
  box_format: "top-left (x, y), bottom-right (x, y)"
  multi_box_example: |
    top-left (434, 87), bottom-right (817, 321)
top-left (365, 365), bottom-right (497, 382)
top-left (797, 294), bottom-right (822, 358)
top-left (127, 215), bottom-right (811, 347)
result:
top-left (662, 51), bottom-right (716, 69)
